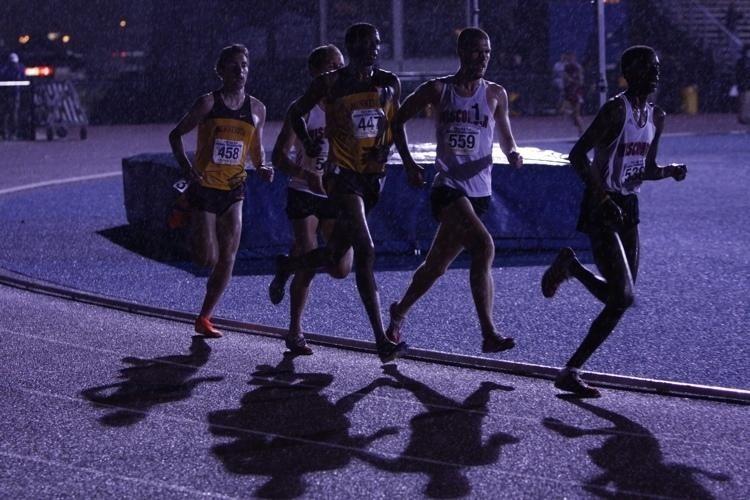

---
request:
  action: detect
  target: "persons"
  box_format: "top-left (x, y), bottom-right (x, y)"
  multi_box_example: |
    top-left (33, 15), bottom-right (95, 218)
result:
top-left (541, 46), bottom-right (688, 399)
top-left (167, 44), bottom-right (275, 338)
top-left (551, 48), bottom-right (586, 137)
top-left (736, 43), bottom-right (750, 123)
top-left (386, 26), bottom-right (524, 354)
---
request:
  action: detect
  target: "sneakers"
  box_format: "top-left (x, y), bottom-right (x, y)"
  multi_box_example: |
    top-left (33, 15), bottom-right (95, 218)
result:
top-left (554, 371), bottom-right (602, 398)
top-left (376, 301), bottom-right (409, 363)
top-left (541, 246), bottom-right (577, 298)
top-left (285, 333), bottom-right (314, 355)
top-left (194, 315), bottom-right (224, 337)
top-left (269, 253), bottom-right (290, 305)
top-left (482, 333), bottom-right (515, 354)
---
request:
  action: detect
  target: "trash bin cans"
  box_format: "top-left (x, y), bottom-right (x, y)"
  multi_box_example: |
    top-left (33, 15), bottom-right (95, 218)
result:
top-left (680, 82), bottom-right (699, 114)
